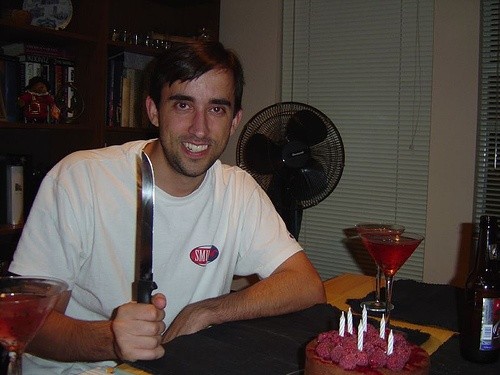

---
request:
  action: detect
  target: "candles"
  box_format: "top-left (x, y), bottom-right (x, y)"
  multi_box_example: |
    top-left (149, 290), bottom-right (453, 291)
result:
top-left (361, 303), bottom-right (371, 331)
top-left (338, 308), bottom-right (346, 337)
top-left (387, 328), bottom-right (394, 354)
top-left (380, 313), bottom-right (386, 338)
top-left (357, 319), bottom-right (365, 352)
top-left (347, 306), bottom-right (353, 336)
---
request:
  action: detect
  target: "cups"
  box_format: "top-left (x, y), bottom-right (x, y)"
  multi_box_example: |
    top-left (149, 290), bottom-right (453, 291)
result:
top-left (111, 27), bottom-right (171, 50)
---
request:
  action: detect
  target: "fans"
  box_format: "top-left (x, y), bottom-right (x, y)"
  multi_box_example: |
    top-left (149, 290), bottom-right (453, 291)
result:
top-left (236, 101), bottom-right (344, 242)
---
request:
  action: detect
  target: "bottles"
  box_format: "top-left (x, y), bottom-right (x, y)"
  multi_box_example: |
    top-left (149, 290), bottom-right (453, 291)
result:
top-left (460, 214), bottom-right (500, 363)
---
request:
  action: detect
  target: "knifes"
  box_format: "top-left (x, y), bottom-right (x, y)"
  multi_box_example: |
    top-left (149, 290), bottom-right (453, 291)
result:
top-left (136, 150), bottom-right (158, 305)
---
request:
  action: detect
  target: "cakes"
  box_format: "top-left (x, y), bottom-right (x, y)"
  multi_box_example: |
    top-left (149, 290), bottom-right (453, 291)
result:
top-left (304, 323), bottom-right (431, 375)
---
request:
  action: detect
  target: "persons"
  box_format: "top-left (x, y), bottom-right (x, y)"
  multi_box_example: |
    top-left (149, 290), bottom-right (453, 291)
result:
top-left (17, 76), bottom-right (57, 125)
top-left (7, 40), bottom-right (327, 375)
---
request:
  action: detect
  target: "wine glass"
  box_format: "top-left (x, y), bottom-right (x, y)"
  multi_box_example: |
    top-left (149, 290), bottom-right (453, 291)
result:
top-left (359, 232), bottom-right (427, 341)
top-left (0, 274), bottom-right (69, 375)
top-left (355, 222), bottom-right (407, 313)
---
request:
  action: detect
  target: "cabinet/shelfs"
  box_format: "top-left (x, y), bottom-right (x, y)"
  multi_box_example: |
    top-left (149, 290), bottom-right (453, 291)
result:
top-left (1, 1), bottom-right (221, 274)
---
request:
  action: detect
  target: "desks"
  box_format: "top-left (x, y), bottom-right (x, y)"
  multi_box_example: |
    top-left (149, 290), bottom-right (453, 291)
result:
top-left (74, 272), bottom-right (499, 374)
